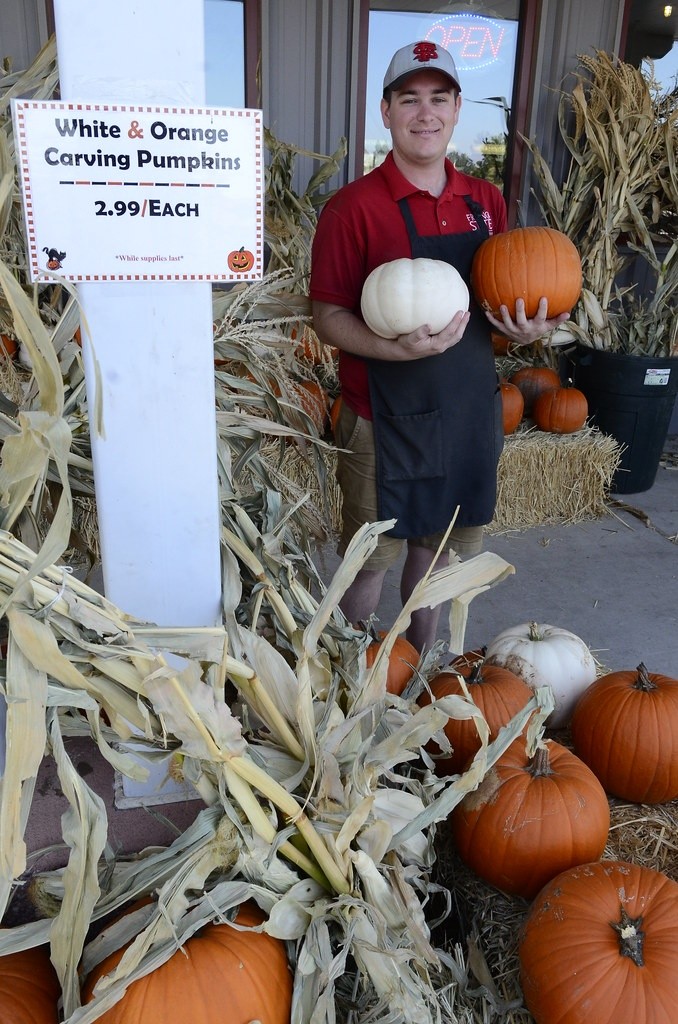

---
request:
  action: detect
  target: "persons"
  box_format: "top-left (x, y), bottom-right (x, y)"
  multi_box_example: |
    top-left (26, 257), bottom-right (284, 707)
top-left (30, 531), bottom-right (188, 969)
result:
top-left (308, 41), bottom-right (571, 658)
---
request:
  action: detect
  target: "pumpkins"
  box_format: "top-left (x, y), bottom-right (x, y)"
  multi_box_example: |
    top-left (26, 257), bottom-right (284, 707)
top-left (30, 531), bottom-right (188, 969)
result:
top-left (0, 228), bottom-right (678, 1024)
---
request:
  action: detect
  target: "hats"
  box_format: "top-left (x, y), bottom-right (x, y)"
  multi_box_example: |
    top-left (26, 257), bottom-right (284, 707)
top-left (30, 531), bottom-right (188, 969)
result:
top-left (383, 41), bottom-right (462, 93)
